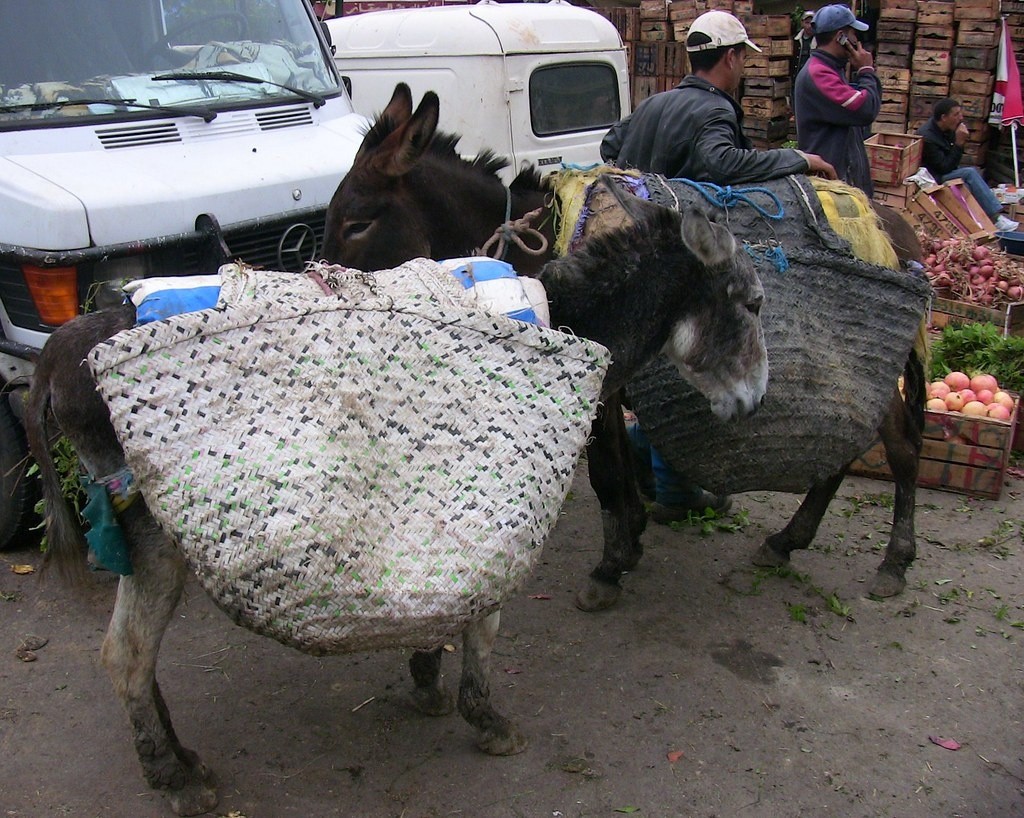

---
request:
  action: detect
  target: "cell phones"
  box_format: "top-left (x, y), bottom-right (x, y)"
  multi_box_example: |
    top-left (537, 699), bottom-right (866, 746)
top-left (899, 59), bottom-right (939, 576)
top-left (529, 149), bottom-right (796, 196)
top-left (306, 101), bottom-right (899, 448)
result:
top-left (838, 33), bottom-right (858, 51)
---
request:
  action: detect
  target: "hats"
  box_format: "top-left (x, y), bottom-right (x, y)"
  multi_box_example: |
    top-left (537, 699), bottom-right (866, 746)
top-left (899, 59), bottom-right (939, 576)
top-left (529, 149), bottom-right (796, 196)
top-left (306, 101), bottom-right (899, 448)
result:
top-left (810, 4), bottom-right (869, 34)
top-left (685, 9), bottom-right (763, 54)
top-left (802, 11), bottom-right (815, 20)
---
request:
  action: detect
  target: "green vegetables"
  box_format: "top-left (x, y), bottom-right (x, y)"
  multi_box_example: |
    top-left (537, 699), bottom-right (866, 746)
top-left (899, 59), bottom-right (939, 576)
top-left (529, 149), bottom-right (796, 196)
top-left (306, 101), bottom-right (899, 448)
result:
top-left (923, 320), bottom-right (1024, 400)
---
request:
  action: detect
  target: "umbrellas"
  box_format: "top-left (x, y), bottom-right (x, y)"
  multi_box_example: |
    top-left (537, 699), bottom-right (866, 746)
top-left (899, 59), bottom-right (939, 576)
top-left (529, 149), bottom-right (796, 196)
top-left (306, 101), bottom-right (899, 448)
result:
top-left (988, 17), bottom-right (1024, 187)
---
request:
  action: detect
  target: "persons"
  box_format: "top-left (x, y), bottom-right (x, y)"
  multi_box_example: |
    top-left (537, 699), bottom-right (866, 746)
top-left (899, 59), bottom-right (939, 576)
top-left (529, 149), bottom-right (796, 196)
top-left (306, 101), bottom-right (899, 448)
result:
top-left (600, 10), bottom-right (838, 183)
top-left (913, 98), bottom-right (1020, 232)
top-left (795, 5), bottom-right (883, 201)
top-left (791, 11), bottom-right (816, 109)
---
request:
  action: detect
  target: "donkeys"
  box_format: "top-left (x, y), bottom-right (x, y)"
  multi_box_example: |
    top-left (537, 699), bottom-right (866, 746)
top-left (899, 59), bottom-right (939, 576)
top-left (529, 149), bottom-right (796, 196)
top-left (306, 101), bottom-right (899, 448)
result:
top-left (31, 176), bottom-right (768, 817)
top-left (322, 85), bottom-right (924, 611)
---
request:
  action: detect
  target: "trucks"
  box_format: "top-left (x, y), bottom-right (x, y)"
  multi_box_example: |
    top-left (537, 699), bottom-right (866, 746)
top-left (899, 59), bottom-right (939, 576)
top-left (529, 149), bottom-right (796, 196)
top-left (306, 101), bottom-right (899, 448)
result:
top-left (0, 0), bottom-right (447, 550)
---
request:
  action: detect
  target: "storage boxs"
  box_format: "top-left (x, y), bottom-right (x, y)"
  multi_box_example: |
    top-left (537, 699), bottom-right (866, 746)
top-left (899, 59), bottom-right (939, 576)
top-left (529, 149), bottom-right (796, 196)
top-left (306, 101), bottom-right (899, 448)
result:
top-left (579, 0), bottom-right (1024, 501)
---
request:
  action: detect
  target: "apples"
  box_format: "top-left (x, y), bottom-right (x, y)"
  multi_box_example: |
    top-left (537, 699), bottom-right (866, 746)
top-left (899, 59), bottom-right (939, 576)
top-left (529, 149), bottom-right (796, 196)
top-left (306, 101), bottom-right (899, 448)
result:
top-left (897, 372), bottom-right (1014, 445)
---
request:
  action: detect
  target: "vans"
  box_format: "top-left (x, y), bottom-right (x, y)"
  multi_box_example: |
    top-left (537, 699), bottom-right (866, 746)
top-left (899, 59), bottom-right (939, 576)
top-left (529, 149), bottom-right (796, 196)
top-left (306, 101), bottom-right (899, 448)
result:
top-left (322, 0), bottom-right (633, 192)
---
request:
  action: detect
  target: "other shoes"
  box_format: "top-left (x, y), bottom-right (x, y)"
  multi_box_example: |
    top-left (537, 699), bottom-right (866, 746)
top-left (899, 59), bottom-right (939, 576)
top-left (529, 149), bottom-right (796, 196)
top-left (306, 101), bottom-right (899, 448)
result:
top-left (650, 490), bottom-right (733, 524)
top-left (635, 475), bottom-right (657, 501)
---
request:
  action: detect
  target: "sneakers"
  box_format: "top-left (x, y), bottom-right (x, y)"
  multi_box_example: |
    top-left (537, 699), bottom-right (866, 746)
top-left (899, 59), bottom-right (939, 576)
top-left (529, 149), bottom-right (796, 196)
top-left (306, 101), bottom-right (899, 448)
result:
top-left (992, 214), bottom-right (1019, 233)
